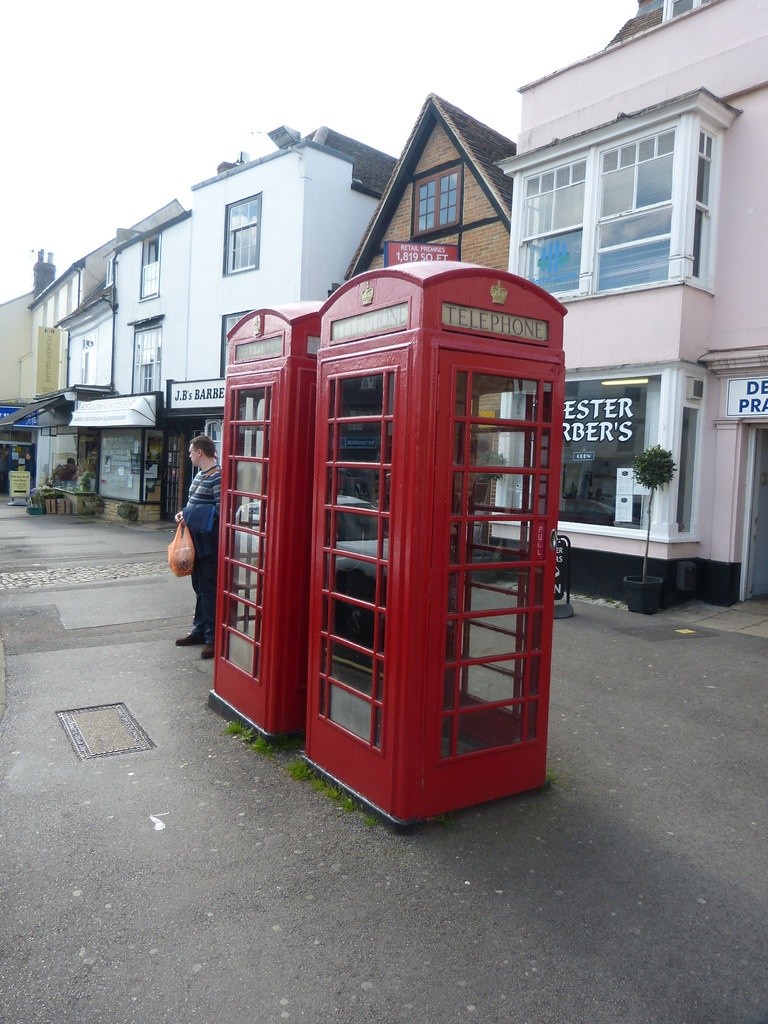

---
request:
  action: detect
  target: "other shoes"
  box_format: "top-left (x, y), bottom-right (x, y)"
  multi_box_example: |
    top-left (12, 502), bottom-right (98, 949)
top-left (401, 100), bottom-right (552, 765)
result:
top-left (201, 643), bottom-right (214, 659)
top-left (175, 634), bottom-right (206, 646)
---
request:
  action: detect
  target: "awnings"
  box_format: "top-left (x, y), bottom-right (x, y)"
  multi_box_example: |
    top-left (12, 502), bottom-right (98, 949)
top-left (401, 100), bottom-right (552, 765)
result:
top-left (0, 395), bottom-right (156, 427)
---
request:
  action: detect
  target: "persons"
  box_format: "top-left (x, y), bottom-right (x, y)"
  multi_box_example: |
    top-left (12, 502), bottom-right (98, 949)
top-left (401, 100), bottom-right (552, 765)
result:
top-left (0, 449), bottom-right (78, 493)
top-left (566, 482), bottom-right (593, 499)
top-left (175, 435), bottom-right (222, 659)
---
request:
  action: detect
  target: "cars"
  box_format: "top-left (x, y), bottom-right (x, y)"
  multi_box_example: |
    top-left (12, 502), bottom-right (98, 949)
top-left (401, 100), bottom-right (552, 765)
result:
top-left (235, 495), bottom-right (459, 662)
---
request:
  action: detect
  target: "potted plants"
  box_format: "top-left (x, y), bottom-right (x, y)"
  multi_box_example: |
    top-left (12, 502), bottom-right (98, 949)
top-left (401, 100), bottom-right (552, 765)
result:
top-left (473, 450), bottom-right (508, 585)
top-left (623, 445), bottom-right (677, 613)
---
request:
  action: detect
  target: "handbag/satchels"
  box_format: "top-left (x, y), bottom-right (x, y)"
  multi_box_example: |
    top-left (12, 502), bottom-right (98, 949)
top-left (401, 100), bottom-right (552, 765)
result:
top-left (167, 521), bottom-right (195, 577)
